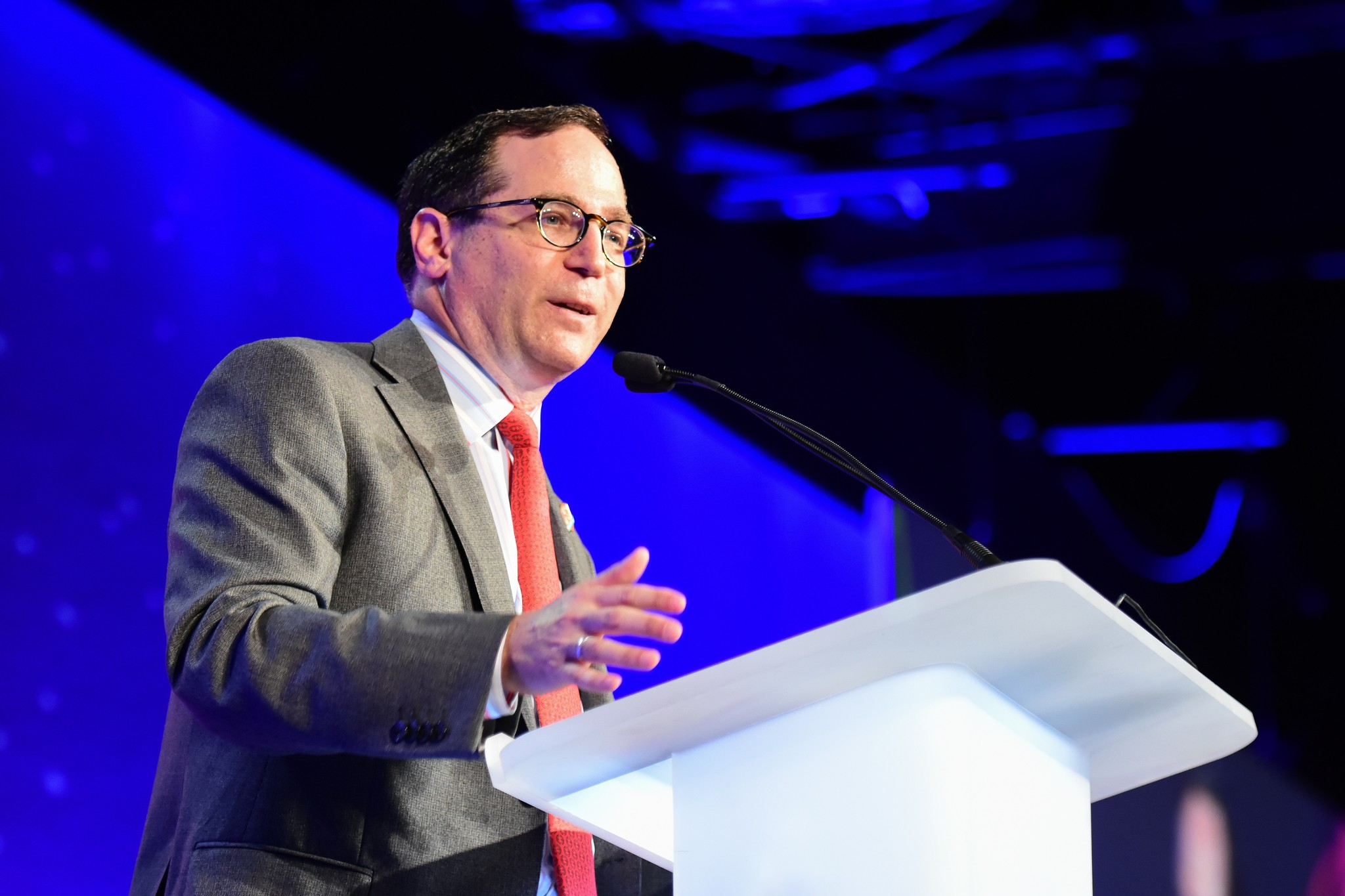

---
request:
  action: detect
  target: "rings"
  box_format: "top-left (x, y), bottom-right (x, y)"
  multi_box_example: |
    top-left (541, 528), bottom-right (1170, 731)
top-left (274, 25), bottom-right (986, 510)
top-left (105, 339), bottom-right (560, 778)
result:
top-left (575, 635), bottom-right (597, 663)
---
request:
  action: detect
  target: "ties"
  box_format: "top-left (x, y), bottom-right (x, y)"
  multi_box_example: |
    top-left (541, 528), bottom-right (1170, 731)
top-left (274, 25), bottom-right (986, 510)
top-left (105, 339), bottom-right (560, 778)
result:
top-left (497, 408), bottom-right (598, 896)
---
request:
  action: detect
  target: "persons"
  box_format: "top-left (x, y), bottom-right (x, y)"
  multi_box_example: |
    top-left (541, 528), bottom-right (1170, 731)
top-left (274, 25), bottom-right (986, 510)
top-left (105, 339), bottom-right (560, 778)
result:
top-left (127, 101), bottom-right (689, 895)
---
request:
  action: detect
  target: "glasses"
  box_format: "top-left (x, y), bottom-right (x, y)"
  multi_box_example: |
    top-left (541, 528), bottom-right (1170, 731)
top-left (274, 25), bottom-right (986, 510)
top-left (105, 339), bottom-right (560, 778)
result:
top-left (445, 196), bottom-right (657, 268)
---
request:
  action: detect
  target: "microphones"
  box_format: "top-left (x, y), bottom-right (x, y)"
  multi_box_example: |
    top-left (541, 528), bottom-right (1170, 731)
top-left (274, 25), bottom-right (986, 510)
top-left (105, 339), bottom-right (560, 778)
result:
top-left (612, 352), bottom-right (1004, 572)
